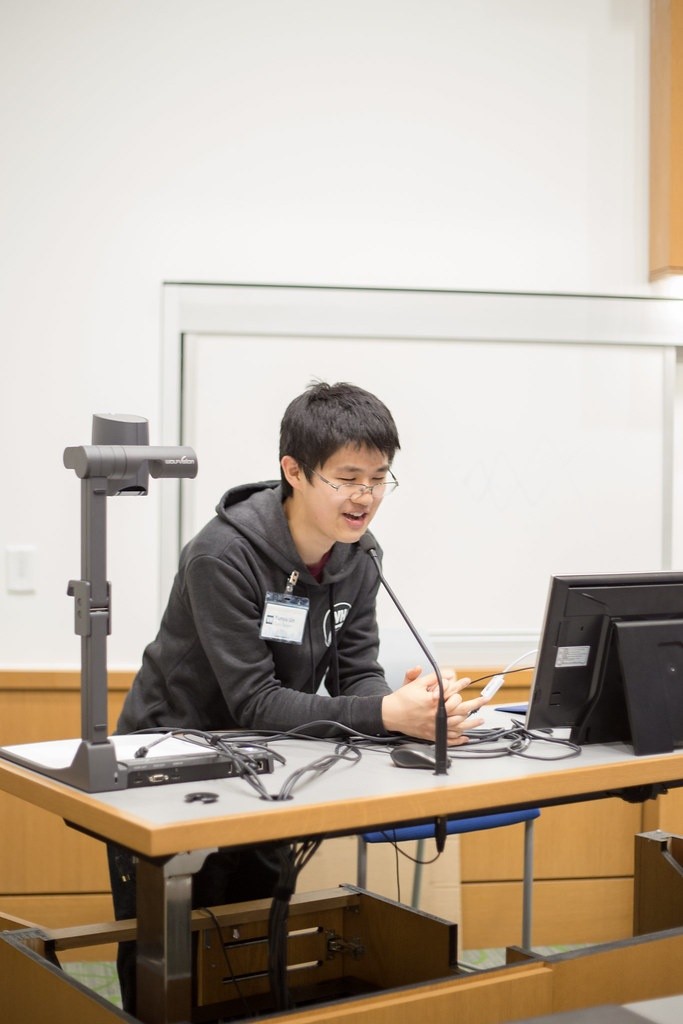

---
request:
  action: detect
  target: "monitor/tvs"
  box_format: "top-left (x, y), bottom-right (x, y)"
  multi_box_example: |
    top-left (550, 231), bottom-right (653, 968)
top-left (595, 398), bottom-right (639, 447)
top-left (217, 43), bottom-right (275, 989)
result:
top-left (526, 572), bottom-right (683, 732)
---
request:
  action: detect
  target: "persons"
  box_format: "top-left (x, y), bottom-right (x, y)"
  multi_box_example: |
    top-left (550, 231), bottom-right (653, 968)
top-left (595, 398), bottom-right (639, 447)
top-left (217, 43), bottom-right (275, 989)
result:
top-left (107, 381), bottom-right (491, 1023)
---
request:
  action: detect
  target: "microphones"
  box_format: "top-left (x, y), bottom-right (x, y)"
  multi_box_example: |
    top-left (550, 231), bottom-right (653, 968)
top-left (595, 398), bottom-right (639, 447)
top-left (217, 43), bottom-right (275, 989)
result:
top-left (360, 533), bottom-right (448, 776)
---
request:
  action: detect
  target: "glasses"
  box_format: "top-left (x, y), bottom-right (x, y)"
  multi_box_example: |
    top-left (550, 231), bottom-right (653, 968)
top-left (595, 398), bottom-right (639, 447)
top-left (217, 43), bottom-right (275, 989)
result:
top-left (300, 459), bottom-right (400, 501)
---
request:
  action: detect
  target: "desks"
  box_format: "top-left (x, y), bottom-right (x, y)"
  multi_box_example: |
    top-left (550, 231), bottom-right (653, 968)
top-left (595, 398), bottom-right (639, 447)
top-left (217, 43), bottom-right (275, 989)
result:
top-left (0, 702), bottom-right (683, 1024)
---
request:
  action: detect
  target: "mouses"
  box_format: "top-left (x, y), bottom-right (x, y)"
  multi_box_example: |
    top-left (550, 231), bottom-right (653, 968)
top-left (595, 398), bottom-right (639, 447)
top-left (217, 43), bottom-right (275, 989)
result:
top-left (390, 744), bottom-right (453, 770)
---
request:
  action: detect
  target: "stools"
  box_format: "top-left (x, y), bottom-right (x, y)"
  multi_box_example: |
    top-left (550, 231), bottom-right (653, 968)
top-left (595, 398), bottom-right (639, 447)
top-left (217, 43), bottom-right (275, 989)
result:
top-left (357, 809), bottom-right (542, 972)
